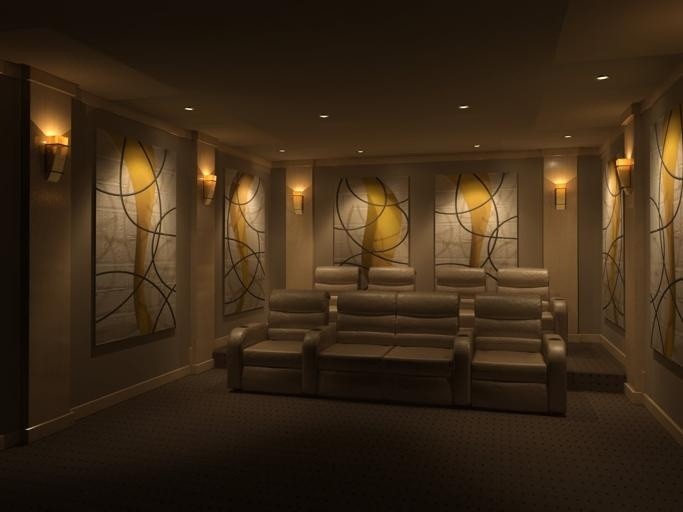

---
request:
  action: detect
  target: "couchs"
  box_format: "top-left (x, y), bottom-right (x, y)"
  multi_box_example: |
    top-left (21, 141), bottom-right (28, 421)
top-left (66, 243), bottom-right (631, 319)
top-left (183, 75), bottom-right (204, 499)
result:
top-left (227, 265), bottom-right (569, 417)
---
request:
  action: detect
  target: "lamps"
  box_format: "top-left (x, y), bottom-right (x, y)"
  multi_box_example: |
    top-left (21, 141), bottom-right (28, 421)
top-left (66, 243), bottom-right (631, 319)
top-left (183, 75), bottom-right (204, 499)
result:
top-left (292, 191), bottom-right (304, 215)
top-left (615, 158), bottom-right (632, 196)
top-left (203, 175), bottom-right (217, 206)
top-left (555, 183), bottom-right (566, 210)
top-left (44, 135), bottom-right (70, 183)
